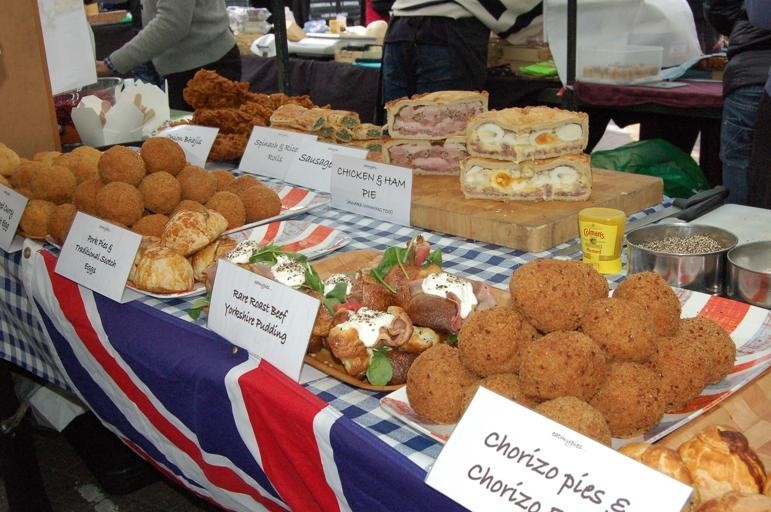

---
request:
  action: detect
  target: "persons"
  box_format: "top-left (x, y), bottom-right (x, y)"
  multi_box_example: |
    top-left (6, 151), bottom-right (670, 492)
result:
top-left (744, 0), bottom-right (771, 211)
top-left (702, 1), bottom-right (771, 204)
top-left (371, 1), bottom-right (545, 131)
top-left (95, 0), bottom-right (241, 113)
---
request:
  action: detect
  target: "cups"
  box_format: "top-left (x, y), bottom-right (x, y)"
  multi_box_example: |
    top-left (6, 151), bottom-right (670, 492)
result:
top-left (337, 15), bottom-right (347, 32)
top-left (302, 19), bottom-right (327, 33)
top-left (329, 20), bottom-right (341, 34)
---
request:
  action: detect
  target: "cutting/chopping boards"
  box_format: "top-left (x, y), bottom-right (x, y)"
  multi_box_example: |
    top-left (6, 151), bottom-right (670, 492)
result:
top-left (319, 168), bottom-right (667, 255)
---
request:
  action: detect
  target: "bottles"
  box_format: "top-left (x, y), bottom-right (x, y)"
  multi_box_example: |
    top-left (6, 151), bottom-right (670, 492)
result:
top-left (577, 206), bottom-right (625, 277)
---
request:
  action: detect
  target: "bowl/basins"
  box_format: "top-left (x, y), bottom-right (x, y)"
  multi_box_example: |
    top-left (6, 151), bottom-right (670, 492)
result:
top-left (54, 77), bottom-right (124, 148)
top-left (624, 222), bottom-right (738, 296)
top-left (576, 43), bottom-right (664, 83)
top-left (725, 240), bottom-right (771, 309)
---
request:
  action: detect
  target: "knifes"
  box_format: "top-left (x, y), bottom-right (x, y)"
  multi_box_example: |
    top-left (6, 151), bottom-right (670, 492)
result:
top-left (624, 186), bottom-right (731, 234)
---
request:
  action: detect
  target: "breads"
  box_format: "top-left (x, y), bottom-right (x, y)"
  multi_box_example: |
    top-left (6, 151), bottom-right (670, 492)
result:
top-left (0, 135), bottom-right (282, 298)
top-left (405, 253), bottom-right (771, 511)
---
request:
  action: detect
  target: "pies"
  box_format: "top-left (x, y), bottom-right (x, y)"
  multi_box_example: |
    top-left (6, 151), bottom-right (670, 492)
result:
top-left (381, 88), bottom-right (490, 178)
top-left (457, 104), bottom-right (594, 204)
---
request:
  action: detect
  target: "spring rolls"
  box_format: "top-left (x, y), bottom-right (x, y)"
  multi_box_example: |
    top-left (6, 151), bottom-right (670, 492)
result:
top-left (267, 102), bottom-right (391, 157)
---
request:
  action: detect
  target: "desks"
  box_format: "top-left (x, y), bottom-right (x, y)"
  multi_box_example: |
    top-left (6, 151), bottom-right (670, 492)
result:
top-left (0, 41), bottom-right (771, 512)
